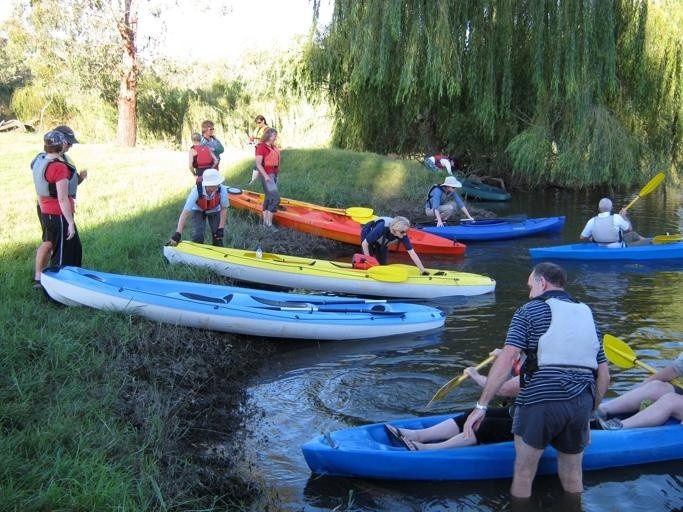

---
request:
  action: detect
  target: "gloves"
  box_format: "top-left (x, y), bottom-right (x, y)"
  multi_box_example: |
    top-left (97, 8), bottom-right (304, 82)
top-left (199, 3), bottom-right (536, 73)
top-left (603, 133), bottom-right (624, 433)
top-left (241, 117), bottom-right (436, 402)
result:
top-left (214, 228), bottom-right (224, 239)
top-left (171, 232), bottom-right (181, 243)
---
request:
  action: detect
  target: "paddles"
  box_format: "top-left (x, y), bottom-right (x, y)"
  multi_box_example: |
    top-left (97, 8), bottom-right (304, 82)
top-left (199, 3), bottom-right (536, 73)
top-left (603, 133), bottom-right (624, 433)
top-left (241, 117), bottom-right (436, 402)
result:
top-left (424, 356), bottom-right (496, 409)
top-left (278, 199), bottom-right (373, 218)
top-left (244, 252), bottom-right (316, 265)
top-left (651, 234), bottom-right (683, 245)
top-left (281, 196), bottom-right (378, 226)
top-left (253, 307), bottom-right (407, 315)
top-left (624, 173), bottom-right (666, 211)
top-left (286, 298), bottom-right (428, 305)
top-left (415, 216), bottom-right (520, 225)
top-left (338, 265), bottom-right (408, 283)
top-left (602, 333), bottom-right (683, 393)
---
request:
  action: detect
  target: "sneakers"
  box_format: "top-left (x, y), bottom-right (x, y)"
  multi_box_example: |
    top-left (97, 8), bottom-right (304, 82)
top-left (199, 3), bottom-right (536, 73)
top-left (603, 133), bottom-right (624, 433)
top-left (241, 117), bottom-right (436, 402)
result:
top-left (31, 278), bottom-right (43, 289)
top-left (590, 406), bottom-right (605, 423)
top-left (266, 224), bottom-right (279, 234)
top-left (262, 221), bottom-right (268, 231)
top-left (597, 417), bottom-right (623, 430)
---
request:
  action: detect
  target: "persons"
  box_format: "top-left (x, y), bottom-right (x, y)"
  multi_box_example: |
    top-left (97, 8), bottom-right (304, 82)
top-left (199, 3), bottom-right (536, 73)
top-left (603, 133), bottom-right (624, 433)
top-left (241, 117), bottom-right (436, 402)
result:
top-left (424, 156), bottom-right (460, 176)
top-left (255, 129), bottom-right (280, 234)
top-left (462, 262), bottom-right (610, 501)
top-left (247, 115), bottom-right (272, 187)
top-left (359, 215), bottom-right (428, 274)
top-left (167, 172), bottom-right (230, 248)
top-left (31, 131), bottom-right (81, 288)
top-left (32, 125), bottom-right (87, 267)
top-left (424, 176), bottom-right (477, 228)
top-left (579, 198), bottom-right (655, 248)
top-left (384, 346), bottom-right (526, 452)
top-left (200, 122), bottom-right (223, 160)
top-left (592, 352), bottom-right (682, 431)
top-left (189, 133), bottom-right (218, 176)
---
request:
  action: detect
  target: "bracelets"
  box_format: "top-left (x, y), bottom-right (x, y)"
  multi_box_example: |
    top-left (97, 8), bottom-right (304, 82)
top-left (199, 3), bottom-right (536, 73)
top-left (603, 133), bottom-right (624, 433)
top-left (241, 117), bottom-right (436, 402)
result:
top-left (477, 402), bottom-right (487, 412)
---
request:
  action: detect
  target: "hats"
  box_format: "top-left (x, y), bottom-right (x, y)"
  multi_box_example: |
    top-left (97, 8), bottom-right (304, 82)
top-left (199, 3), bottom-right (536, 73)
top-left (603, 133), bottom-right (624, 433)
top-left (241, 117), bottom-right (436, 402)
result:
top-left (53, 126), bottom-right (79, 145)
top-left (202, 169), bottom-right (225, 186)
top-left (440, 176), bottom-right (463, 188)
top-left (43, 131), bottom-right (67, 146)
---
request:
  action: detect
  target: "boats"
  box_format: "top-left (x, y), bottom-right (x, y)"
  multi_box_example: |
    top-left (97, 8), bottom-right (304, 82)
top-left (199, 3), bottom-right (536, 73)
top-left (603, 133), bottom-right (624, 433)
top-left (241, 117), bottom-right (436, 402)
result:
top-left (419, 214), bottom-right (566, 239)
top-left (299, 409), bottom-right (683, 481)
top-left (528, 236), bottom-right (683, 265)
top-left (460, 180), bottom-right (511, 204)
top-left (34, 264), bottom-right (448, 343)
top-left (217, 186), bottom-right (467, 256)
top-left (160, 237), bottom-right (496, 300)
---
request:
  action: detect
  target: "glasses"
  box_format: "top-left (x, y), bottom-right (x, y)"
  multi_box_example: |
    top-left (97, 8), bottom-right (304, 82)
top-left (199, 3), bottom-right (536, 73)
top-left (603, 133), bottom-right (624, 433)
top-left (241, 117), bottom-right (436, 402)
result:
top-left (63, 141), bottom-right (73, 146)
top-left (256, 120), bottom-right (262, 124)
top-left (208, 128), bottom-right (214, 130)
top-left (449, 187), bottom-right (455, 189)
top-left (396, 230), bottom-right (405, 234)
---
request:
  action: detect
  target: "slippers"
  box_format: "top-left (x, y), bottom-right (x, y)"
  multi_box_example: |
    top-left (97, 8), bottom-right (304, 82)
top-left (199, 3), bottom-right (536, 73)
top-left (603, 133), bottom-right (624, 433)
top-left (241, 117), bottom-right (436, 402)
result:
top-left (383, 423), bottom-right (404, 442)
top-left (401, 439), bottom-right (419, 451)
